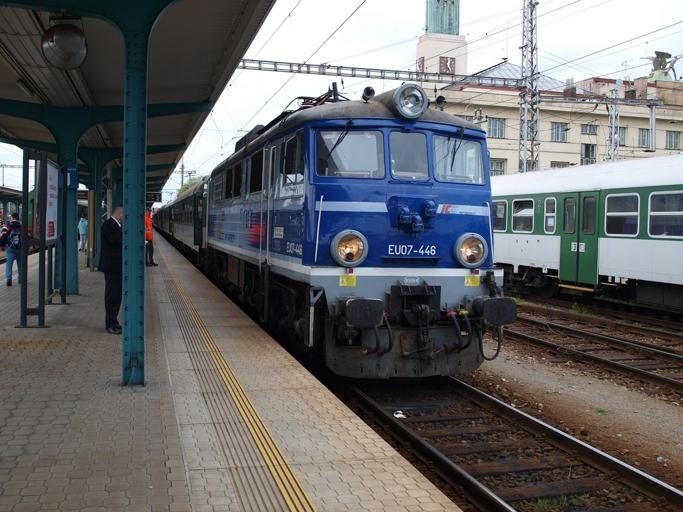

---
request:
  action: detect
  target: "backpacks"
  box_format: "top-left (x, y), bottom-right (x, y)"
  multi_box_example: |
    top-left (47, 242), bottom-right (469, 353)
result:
top-left (8, 222), bottom-right (21, 249)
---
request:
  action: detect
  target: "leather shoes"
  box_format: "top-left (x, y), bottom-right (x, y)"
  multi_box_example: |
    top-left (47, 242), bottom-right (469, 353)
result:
top-left (146, 263), bottom-right (158, 267)
top-left (106, 323), bottom-right (122, 334)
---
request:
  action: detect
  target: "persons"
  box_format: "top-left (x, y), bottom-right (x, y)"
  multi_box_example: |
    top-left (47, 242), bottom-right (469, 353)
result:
top-left (0, 212), bottom-right (22, 286)
top-left (145, 207), bottom-right (158, 266)
top-left (97, 204), bottom-right (122, 335)
top-left (77, 212), bottom-right (89, 251)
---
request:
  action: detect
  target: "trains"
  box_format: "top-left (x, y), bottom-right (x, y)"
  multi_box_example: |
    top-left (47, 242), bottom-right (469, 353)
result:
top-left (488, 151), bottom-right (683, 317)
top-left (149, 79), bottom-right (520, 385)
